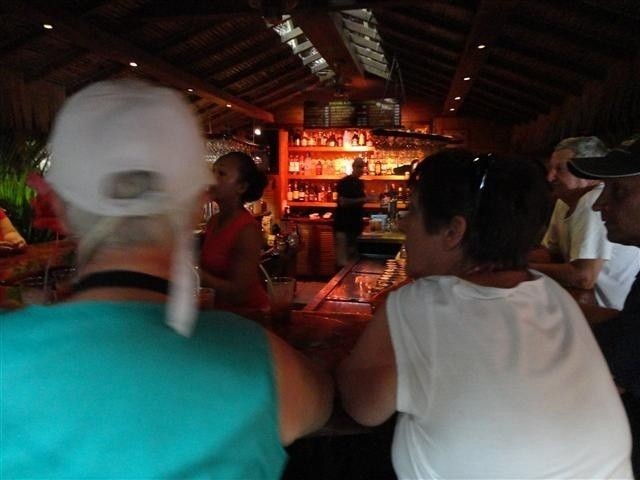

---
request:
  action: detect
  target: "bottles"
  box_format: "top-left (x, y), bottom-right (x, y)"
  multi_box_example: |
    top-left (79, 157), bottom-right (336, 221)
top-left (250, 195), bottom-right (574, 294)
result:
top-left (294, 129), bottom-right (366, 146)
top-left (287, 180), bottom-right (338, 201)
top-left (369, 155), bottom-right (381, 175)
top-left (288, 152), bottom-right (369, 175)
top-left (380, 182), bottom-right (411, 204)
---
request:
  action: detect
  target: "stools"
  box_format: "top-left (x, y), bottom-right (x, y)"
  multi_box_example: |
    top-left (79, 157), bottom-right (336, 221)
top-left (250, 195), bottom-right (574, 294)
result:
top-left (289, 308), bottom-right (377, 442)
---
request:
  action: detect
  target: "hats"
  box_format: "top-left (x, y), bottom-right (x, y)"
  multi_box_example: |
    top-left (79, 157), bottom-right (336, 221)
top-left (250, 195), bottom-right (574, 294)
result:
top-left (566, 137), bottom-right (639, 181)
top-left (43, 78), bottom-right (213, 342)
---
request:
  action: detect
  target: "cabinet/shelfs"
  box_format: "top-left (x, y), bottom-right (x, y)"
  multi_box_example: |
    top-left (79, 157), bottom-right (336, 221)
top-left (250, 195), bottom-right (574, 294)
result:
top-left (281, 123), bottom-right (450, 213)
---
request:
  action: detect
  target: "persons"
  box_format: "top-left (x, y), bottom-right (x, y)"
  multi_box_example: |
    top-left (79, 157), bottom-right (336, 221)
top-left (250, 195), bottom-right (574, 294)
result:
top-left (0, 206), bottom-right (29, 255)
top-left (523, 135), bottom-right (640, 312)
top-left (333, 158), bottom-right (381, 247)
top-left (194, 150), bottom-right (273, 309)
top-left (0, 75), bottom-right (335, 480)
top-left (333, 144), bottom-right (634, 480)
top-left (566, 133), bottom-right (640, 480)
